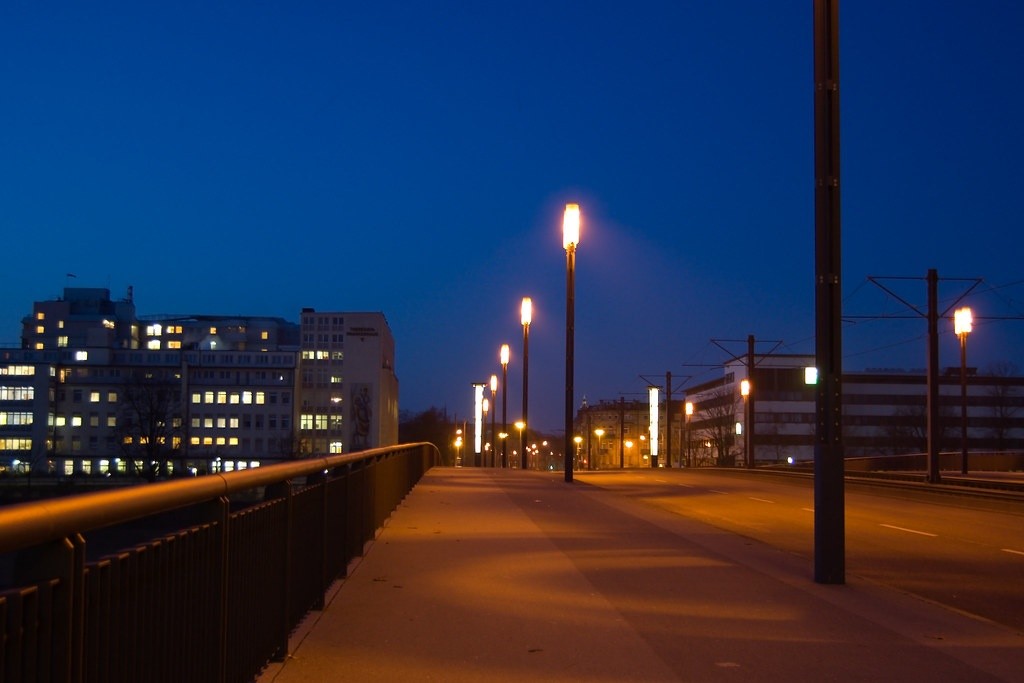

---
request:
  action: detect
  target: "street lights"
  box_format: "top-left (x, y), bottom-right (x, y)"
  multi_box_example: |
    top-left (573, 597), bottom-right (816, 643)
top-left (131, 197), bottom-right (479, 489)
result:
top-left (561, 201), bottom-right (582, 483)
top-left (739, 378), bottom-right (750, 466)
top-left (482, 397), bottom-right (489, 467)
top-left (520, 295), bottom-right (533, 471)
top-left (593, 429), bottom-right (606, 471)
top-left (952, 306), bottom-right (976, 477)
top-left (573, 435), bottom-right (583, 472)
top-left (490, 374), bottom-right (498, 469)
top-left (684, 400), bottom-right (694, 469)
top-left (500, 342), bottom-right (510, 466)
